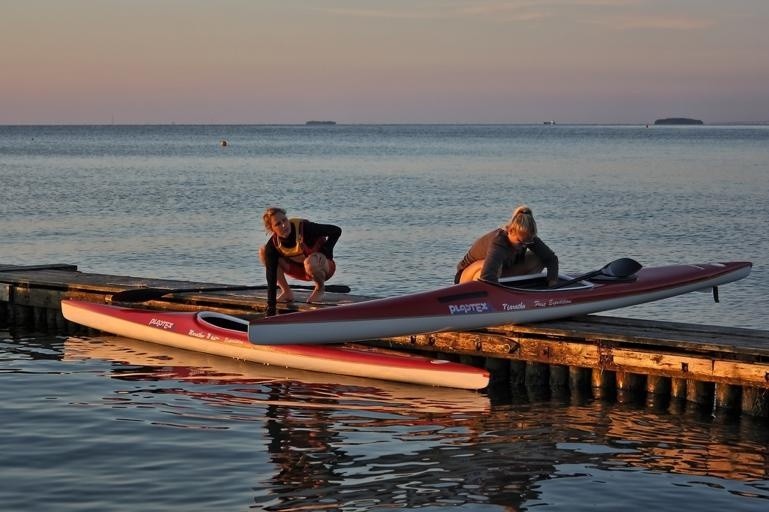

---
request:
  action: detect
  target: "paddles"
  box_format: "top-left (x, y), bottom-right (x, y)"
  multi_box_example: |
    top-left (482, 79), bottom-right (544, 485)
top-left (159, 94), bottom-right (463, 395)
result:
top-left (112, 285), bottom-right (351, 302)
top-left (547, 257), bottom-right (642, 289)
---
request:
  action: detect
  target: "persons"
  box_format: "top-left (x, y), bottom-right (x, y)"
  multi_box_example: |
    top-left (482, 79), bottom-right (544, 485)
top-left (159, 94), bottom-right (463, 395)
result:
top-left (258, 208), bottom-right (341, 317)
top-left (454, 205), bottom-right (558, 289)
